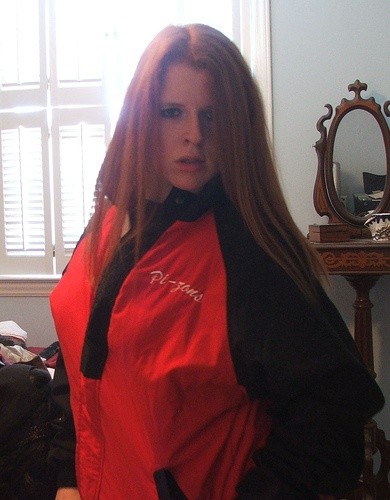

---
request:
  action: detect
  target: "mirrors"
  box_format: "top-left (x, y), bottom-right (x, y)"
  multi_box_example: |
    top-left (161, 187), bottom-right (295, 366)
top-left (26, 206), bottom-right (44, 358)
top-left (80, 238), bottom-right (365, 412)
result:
top-left (311, 79), bottom-right (390, 240)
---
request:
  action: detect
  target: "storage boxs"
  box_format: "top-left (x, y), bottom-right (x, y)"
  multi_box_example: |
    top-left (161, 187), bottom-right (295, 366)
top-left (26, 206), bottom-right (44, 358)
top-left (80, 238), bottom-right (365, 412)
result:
top-left (309, 223), bottom-right (352, 243)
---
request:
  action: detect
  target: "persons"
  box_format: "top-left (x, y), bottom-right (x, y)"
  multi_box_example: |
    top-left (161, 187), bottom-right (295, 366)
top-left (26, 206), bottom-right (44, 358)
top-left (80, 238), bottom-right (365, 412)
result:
top-left (45, 24), bottom-right (385, 500)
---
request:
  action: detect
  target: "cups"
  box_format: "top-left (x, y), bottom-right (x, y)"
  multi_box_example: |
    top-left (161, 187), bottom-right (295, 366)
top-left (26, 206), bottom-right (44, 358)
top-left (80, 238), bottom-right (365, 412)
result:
top-left (365, 212), bottom-right (390, 242)
